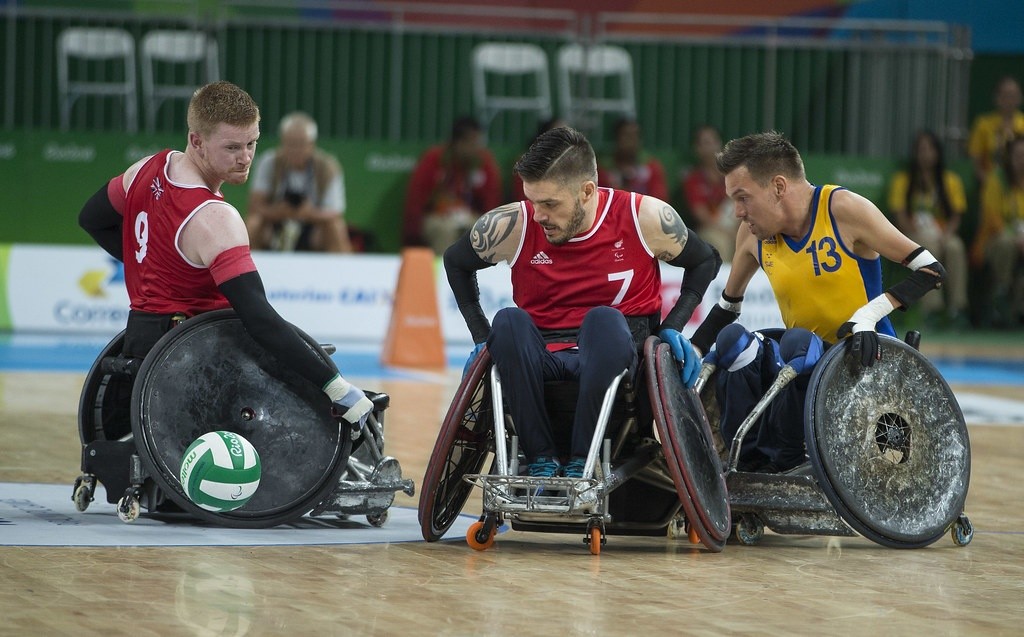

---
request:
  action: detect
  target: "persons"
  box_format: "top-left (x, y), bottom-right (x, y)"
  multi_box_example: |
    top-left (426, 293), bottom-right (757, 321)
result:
top-left (968, 74), bottom-right (1024, 181)
top-left (680, 126), bottom-right (743, 264)
top-left (886, 131), bottom-right (968, 330)
top-left (688, 131), bottom-right (948, 474)
top-left (443, 125), bottom-right (723, 509)
top-left (243, 111), bottom-right (352, 253)
top-left (598, 119), bottom-right (670, 202)
top-left (402, 117), bottom-right (502, 256)
top-left (79, 81), bottom-right (374, 441)
top-left (513, 119), bottom-right (568, 201)
top-left (971, 137), bottom-right (1024, 329)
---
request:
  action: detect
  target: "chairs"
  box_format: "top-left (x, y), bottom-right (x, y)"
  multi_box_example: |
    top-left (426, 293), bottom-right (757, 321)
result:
top-left (558, 45), bottom-right (637, 142)
top-left (473, 41), bottom-right (554, 138)
top-left (59, 25), bottom-right (137, 135)
top-left (141, 29), bottom-right (218, 139)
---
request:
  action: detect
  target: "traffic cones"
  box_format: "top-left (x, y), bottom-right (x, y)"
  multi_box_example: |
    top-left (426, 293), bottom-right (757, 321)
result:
top-left (381, 245), bottom-right (450, 374)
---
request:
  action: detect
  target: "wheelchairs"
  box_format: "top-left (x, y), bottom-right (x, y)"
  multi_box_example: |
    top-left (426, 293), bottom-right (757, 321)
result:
top-left (418, 333), bottom-right (733, 556)
top-left (693, 331), bottom-right (974, 548)
top-left (71, 309), bottom-right (415, 527)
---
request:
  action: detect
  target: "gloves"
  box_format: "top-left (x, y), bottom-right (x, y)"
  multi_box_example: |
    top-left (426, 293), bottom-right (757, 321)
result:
top-left (322, 373), bottom-right (374, 440)
top-left (836, 293), bottom-right (894, 367)
top-left (462, 342), bottom-right (487, 382)
top-left (659, 328), bottom-right (702, 388)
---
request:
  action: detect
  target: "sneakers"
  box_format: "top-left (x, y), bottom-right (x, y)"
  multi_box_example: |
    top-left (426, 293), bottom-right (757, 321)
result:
top-left (557, 456), bottom-right (587, 497)
top-left (515, 456), bottom-right (564, 496)
